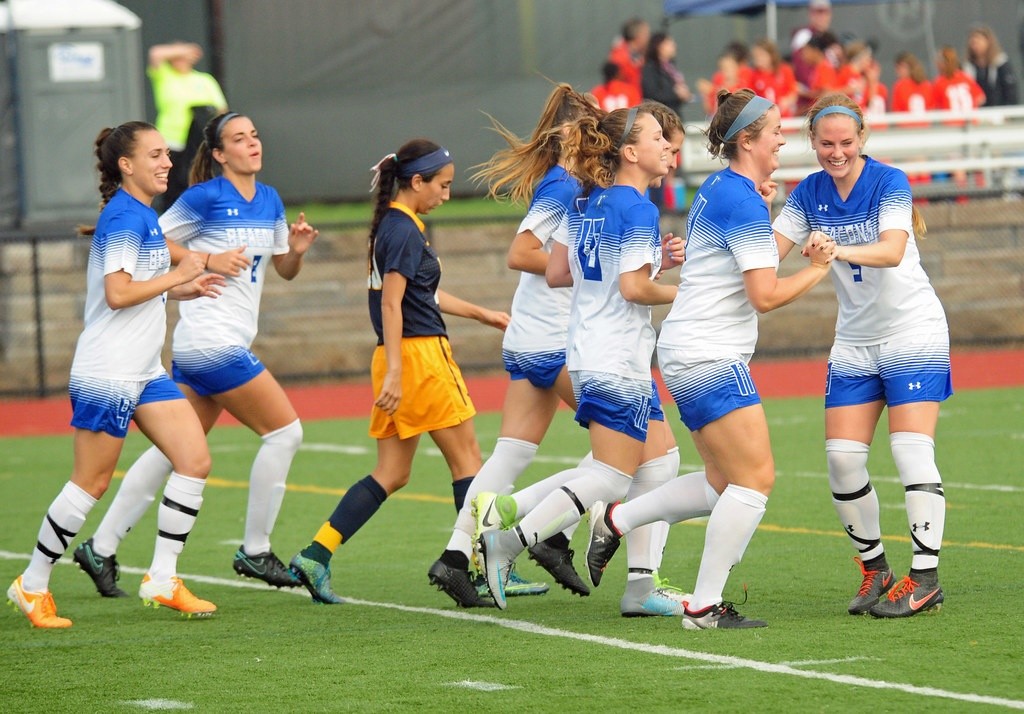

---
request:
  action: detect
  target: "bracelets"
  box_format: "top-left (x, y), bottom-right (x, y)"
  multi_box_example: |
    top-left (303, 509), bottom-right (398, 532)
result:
top-left (205, 253), bottom-right (211, 270)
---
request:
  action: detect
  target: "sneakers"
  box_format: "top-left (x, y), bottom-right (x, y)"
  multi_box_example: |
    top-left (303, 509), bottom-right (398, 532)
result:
top-left (469, 491), bottom-right (517, 579)
top-left (653, 574), bottom-right (693, 606)
top-left (288, 548), bottom-right (346, 605)
top-left (585, 498), bottom-right (623, 588)
top-left (619, 585), bottom-right (685, 616)
top-left (6, 575), bottom-right (72, 628)
top-left (138, 572), bottom-right (217, 619)
top-left (472, 571), bottom-right (549, 598)
top-left (526, 539), bottom-right (591, 598)
top-left (871, 571), bottom-right (944, 618)
top-left (681, 584), bottom-right (768, 631)
top-left (848, 556), bottom-right (896, 614)
top-left (233, 545), bottom-right (303, 589)
top-left (71, 536), bottom-right (129, 599)
top-left (428, 558), bottom-right (494, 608)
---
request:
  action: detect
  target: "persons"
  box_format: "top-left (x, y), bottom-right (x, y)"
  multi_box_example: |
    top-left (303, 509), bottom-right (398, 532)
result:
top-left (71, 113), bottom-right (303, 597)
top-left (7, 121), bottom-right (217, 630)
top-left (771, 94), bottom-right (954, 617)
top-left (583, 89), bottom-right (834, 626)
top-left (591, 0), bottom-right (1024, 208)
top-left (429, 82), bottom-right (692, 618)
top-left (145, 31), bottom-right (230, 215)
top-left (288, 141), bottom-right (550, 603)
